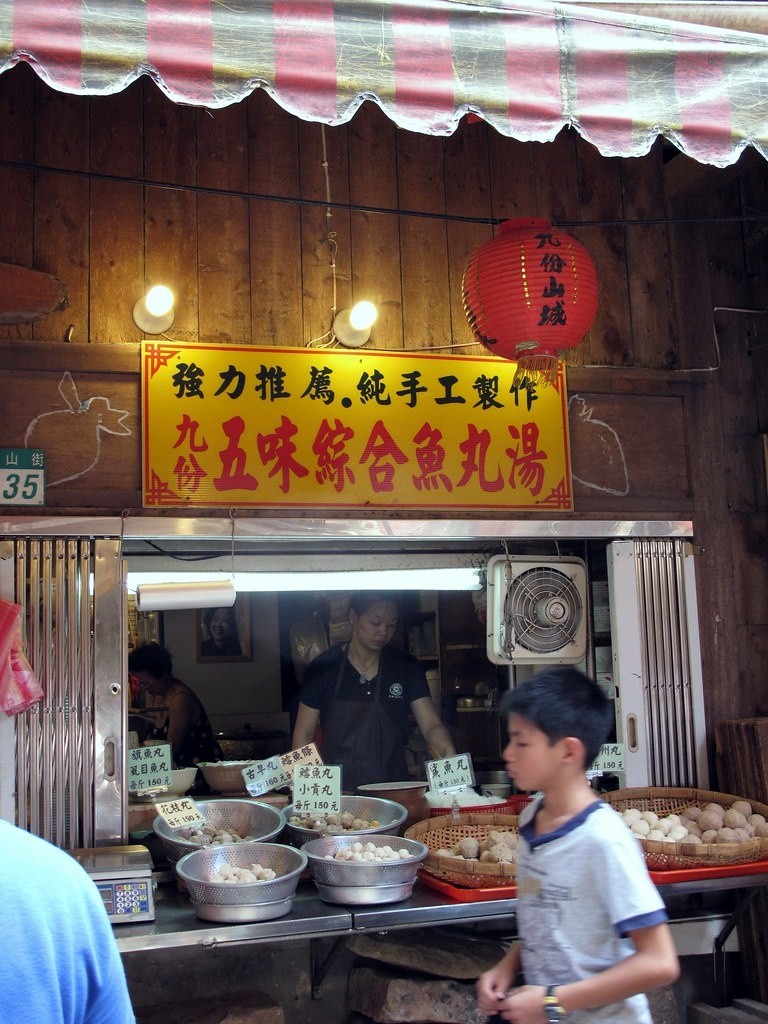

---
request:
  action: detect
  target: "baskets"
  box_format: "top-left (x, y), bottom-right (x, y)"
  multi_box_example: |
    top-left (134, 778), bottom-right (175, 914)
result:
top-left (404, 810), bottom-right (530, 887)
top-left (429, 795), bottom-right (537, 816)
top-left (594, 787), bottom-right (768, 869)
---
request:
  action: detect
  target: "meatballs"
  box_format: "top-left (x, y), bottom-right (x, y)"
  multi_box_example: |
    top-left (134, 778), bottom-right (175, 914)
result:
top-left (207, 864), bottom-right (277, 884)
top-left (179, 823), bottom-right (256, 845)
top-left (616, 801), bottom-right (768, 862)
top-left (323, 842), bottom-right (415, 862)
top-left (434, 831), bottom-right (519, 863)
top-left (289, 810), bottom-right (383, 832)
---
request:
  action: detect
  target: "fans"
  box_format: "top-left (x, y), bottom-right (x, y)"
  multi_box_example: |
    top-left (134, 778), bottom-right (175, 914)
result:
top-left (485, 554), bottom-right (587, 667)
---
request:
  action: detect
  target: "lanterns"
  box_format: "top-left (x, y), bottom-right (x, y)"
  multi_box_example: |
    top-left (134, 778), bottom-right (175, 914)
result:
top-left (461, 216), bottom-right (600, 388)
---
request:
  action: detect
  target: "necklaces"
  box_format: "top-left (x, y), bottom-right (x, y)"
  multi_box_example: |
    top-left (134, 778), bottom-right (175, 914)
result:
top-left (350, 644), bottom-right (378, 684)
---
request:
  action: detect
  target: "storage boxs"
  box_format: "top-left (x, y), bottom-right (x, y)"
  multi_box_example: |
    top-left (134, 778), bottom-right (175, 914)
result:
top-left (331, 622), bottom-right (353, 644)
top-left (328, 596), bottom-right (349, 622)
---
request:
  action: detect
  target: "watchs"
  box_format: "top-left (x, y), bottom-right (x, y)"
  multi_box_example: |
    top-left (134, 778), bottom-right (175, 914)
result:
top-left (542, 984), bottom-right (568, 1024)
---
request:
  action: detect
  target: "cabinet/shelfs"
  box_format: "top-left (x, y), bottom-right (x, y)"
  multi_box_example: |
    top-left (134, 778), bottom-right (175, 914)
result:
top-left (414, 590), bottom-right (508, 782)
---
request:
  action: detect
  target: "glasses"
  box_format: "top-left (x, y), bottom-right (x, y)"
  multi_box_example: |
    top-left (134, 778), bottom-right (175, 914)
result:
top-left (136, 679), bottom-right (154, 689)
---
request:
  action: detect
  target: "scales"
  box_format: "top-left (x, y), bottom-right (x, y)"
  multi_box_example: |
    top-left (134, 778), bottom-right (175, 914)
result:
top-left (63, 845), bottom-right (158, 923)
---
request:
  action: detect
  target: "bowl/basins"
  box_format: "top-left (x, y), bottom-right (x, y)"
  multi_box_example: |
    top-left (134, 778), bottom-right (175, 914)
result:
top-left (480, 783), bottom-right (511, 799)
top-left (128, 767), bottom-right (199, 803)
top-left (196, 760), bottom-right (255, 796)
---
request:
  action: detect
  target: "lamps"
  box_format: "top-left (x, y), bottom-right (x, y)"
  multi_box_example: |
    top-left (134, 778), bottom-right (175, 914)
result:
top-left (332, 300), bottom-right (378, 349)
top-left (77, 549), bottom-right (493, 595)
top-left (131, 283), bottom-right (176, 336)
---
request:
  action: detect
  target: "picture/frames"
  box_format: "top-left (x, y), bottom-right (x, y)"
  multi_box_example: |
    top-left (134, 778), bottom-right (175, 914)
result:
top-left (195, 592), bottom-right (251, 664)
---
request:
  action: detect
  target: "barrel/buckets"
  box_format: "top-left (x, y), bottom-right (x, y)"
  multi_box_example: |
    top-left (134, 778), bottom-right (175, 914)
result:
top-left (356, 780), bottom-right (431, 838)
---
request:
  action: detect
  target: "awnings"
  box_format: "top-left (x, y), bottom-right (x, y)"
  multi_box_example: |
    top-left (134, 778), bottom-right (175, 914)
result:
top-left (0, 0), bottom-right (768, 169)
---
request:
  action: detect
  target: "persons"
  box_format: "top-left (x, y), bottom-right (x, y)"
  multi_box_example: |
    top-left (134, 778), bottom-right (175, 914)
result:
top-left (475, 665), bottom-right (680, 1024)
top-left (0, 819), bottom-right (136, 1024)
top-left (128, 641), bottom-right (223, 796)
top-left (199, 606), bottom-right (243, 655)
top-left (292, 590), bottom-right (457, 792)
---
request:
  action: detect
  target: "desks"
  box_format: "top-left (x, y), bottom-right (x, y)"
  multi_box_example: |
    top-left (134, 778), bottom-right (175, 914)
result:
top-left (310, 876), bottom-right (757, 1000)
top-left (110, 885), bottom-right (353, 1003)
top-left (127, 792), bottom-right (290, 831)
